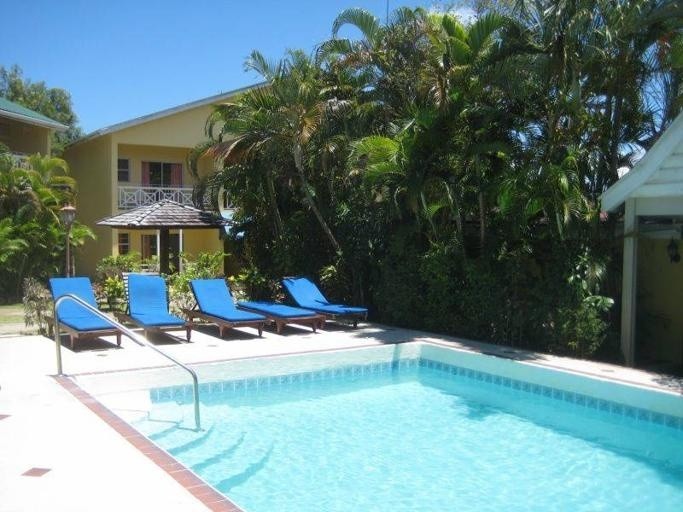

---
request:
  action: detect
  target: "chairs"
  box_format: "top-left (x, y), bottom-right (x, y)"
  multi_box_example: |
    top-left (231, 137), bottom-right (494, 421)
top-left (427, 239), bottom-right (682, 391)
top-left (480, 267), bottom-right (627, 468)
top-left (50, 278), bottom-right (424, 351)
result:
top-left (180, 278), bottom-right (268, 340)
top-left (235, 300), bottom-right (327, 335)
top-left (113, 274), bottom-right (194, 343)
top-left (48, 275), bottom-right (125, 347)
top-left (280, 275), bottom-right (369, 330)
